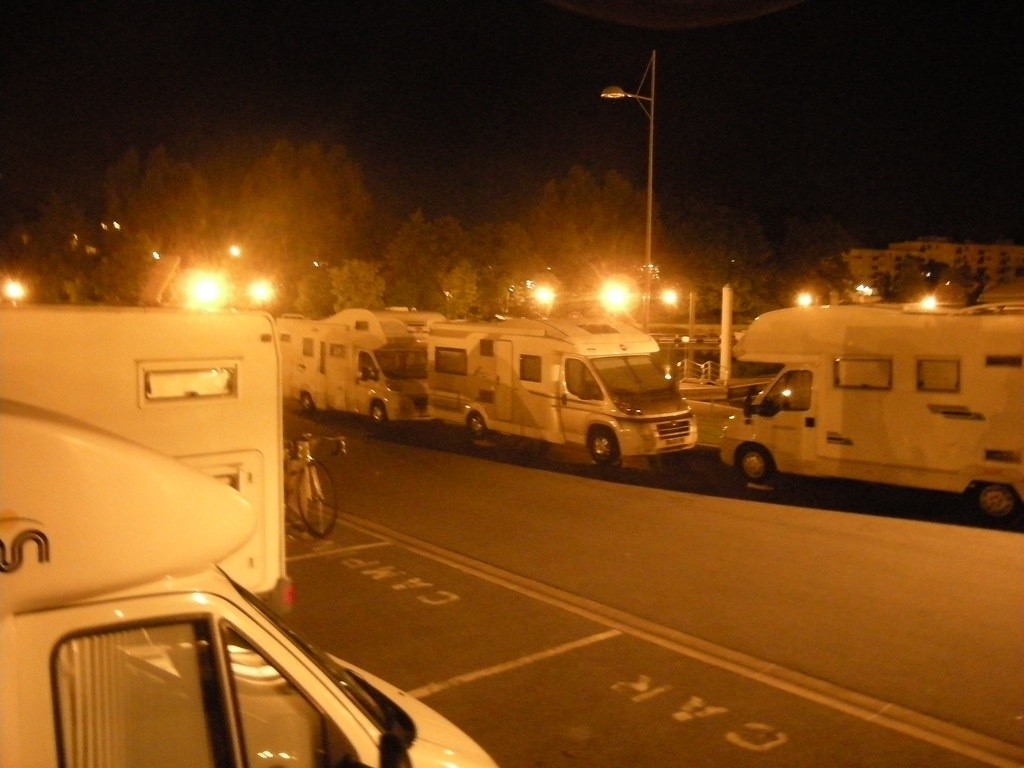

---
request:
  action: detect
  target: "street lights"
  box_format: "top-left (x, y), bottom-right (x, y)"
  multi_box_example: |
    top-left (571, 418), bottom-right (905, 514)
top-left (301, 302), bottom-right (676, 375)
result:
top-left (601, 87), bottom-right (654, 334)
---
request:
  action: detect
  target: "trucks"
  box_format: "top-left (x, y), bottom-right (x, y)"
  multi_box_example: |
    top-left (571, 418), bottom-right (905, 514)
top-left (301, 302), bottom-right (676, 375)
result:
top-left (1, 308), bottom-right (287, 596)
top-left (425, 317), bottom-right (699, 466)
top-left (1, 398), bottom-right (499, 768)
top-left (719, 301), bottom-right (1024, 522)
top-left (275, 308), bottom-right (437, 424)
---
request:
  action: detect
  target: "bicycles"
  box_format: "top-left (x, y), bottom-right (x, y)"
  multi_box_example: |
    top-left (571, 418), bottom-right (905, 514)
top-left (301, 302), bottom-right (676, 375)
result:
top-left (284, 433), bottom-right (348, 539)
